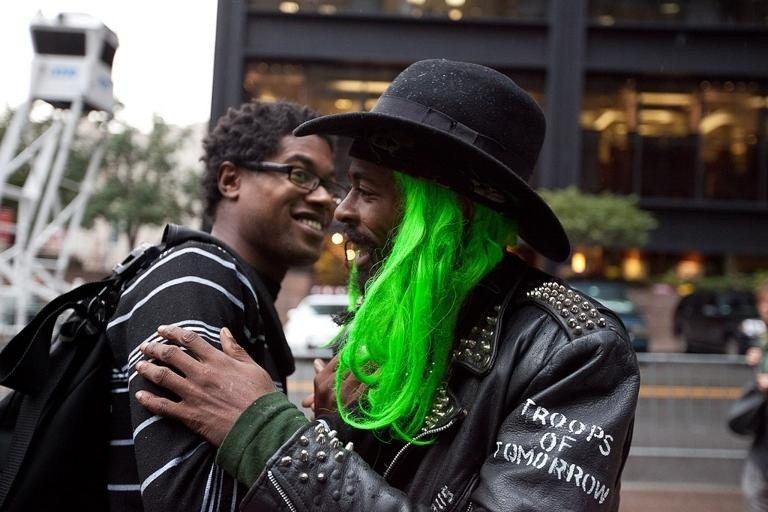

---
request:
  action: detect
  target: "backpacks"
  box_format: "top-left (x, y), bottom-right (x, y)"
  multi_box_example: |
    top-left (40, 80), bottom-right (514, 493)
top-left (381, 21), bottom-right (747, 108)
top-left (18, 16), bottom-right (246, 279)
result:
top-left (0, 222), bottom-right (294, 509)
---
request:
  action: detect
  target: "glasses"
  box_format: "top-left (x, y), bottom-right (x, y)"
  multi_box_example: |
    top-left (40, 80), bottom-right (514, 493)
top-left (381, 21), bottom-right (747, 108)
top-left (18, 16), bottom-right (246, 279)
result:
top-left (240, 157), bottom-right (348, 207)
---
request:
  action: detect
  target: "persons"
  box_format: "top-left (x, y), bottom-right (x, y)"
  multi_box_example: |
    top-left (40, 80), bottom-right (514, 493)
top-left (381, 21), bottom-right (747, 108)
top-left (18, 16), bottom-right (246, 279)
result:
top-left (132, 58), bottom-right (641, 512)
top-left (730, 280), bottom-right (768, 512)
top-left (0, 98), bottom-right (339, 512)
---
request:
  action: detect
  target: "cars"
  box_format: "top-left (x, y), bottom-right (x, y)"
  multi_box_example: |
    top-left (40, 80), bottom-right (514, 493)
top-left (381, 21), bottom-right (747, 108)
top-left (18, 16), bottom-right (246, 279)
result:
top-left (568, 279), bottom-right (650, 352)
top-left (672, 288), bottom-right (768, 355)
top-left (283, 293), bottom-right (349, 359)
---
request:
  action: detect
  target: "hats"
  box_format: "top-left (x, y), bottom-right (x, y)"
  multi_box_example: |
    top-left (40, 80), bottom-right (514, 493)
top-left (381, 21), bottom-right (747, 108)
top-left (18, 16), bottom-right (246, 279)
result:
top-left (293, 59), bottom-right (570, 264)
